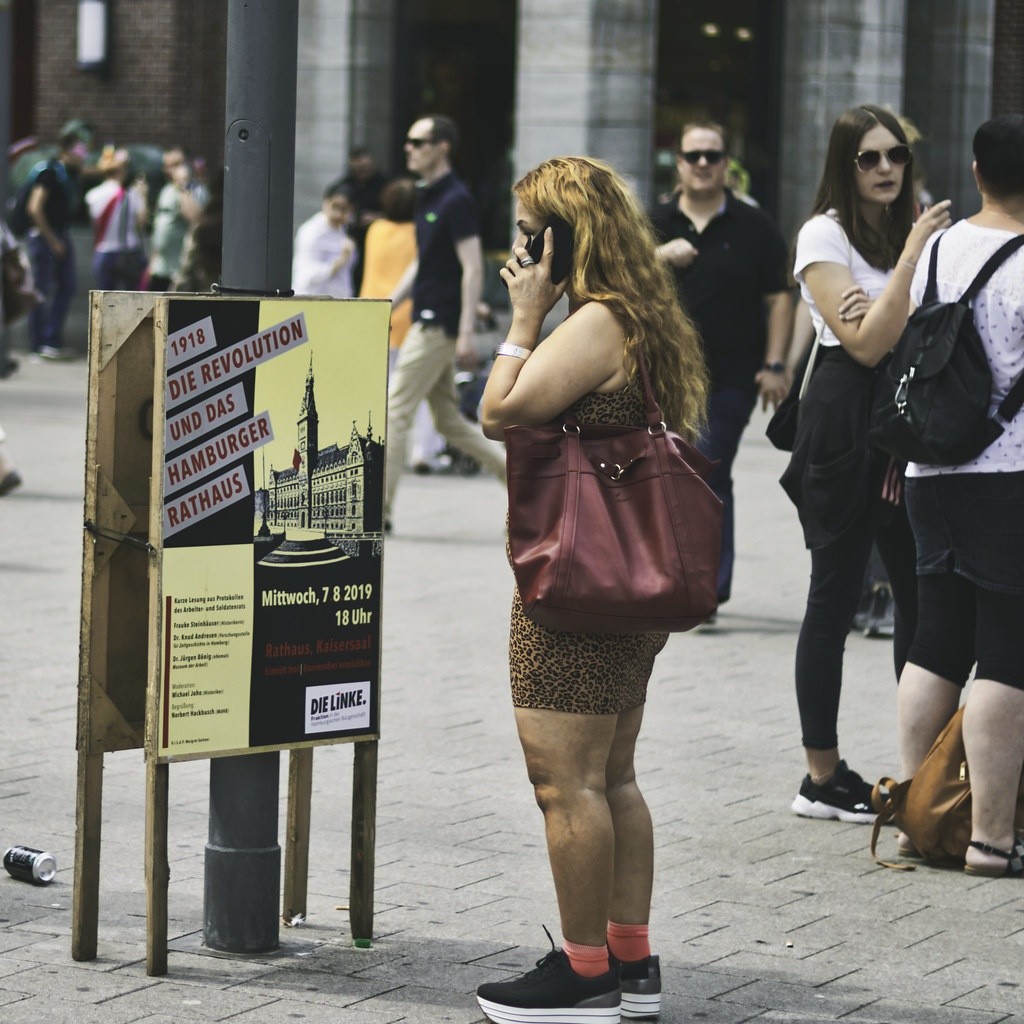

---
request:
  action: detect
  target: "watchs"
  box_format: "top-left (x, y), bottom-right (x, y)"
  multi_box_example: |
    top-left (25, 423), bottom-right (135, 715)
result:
top-left (763, 361), bottom-right (786, 373)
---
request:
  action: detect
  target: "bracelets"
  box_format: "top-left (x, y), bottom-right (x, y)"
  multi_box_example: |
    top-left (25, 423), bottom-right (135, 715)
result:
top-left (899, 261), bottom-right (914, 271)
top-left (495, 341), bottom-right (532, 360)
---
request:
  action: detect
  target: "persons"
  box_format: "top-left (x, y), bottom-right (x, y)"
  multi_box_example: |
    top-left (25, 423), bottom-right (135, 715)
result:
top-left (292, 114), bottom-right (507, 533)
top-left (473, 158), bottom-right (710, 1023)
top-left (1, 121), bottom-right (224, 494)
top-left (645, 102), bottom-right (1024, 878)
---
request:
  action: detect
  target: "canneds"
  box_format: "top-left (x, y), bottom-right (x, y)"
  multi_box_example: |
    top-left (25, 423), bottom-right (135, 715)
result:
top-left (4, 845), bottom-right (56, 885)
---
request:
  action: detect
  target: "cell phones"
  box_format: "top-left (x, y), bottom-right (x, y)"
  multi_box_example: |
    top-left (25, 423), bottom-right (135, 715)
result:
top-left (502, 214), bottom-right (577, 289)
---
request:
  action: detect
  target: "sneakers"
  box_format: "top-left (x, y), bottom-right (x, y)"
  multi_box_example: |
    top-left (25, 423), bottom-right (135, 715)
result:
top-left (476, 947), bottom-right (661, 1024)
top-left (793, 759), bottom-right (894, 824)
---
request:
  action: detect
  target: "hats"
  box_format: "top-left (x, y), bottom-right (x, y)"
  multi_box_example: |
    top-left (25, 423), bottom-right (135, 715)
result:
top-left (59, 120), bottom-right (97, 144)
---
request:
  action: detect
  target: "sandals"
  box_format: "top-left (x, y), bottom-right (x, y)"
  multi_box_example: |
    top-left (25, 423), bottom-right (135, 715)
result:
top-left (894, 829), bottom-right (918, 856)
top-left (965, 838), bottom-right (1023, 875)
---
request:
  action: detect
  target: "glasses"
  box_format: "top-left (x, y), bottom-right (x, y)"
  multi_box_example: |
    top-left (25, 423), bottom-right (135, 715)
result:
top-left (406, 138), bottom-right (431, 148)
top-left (854, 145), bottom-right (912, 173)
top-left (678, 150), bottom-right (726, 166)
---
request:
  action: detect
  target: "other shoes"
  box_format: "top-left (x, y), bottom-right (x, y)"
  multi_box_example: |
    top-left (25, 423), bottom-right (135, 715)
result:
top-left (39, 344), bottom-right (80, 362)
top-left (690, 612), bottom-right (718, 633)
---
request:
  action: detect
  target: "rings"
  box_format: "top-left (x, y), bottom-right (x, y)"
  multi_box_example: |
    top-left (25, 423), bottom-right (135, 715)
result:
top-left (519, 256), bottom-right (536, 268)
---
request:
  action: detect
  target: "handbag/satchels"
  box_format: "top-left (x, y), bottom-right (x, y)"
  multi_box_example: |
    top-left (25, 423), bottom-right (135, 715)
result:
top-left (1, 246), bottom-right (38, 319)
top-left (871, 702), bottom-right (1024, 870)
top-left (503, 323), bottom-right (719, 634)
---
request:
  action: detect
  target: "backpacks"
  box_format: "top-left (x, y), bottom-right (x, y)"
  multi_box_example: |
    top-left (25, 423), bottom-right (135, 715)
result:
top-left (870, 229), bottom-right (1024, 463)
top-left (13, 159), bottom-right (68, 231)
